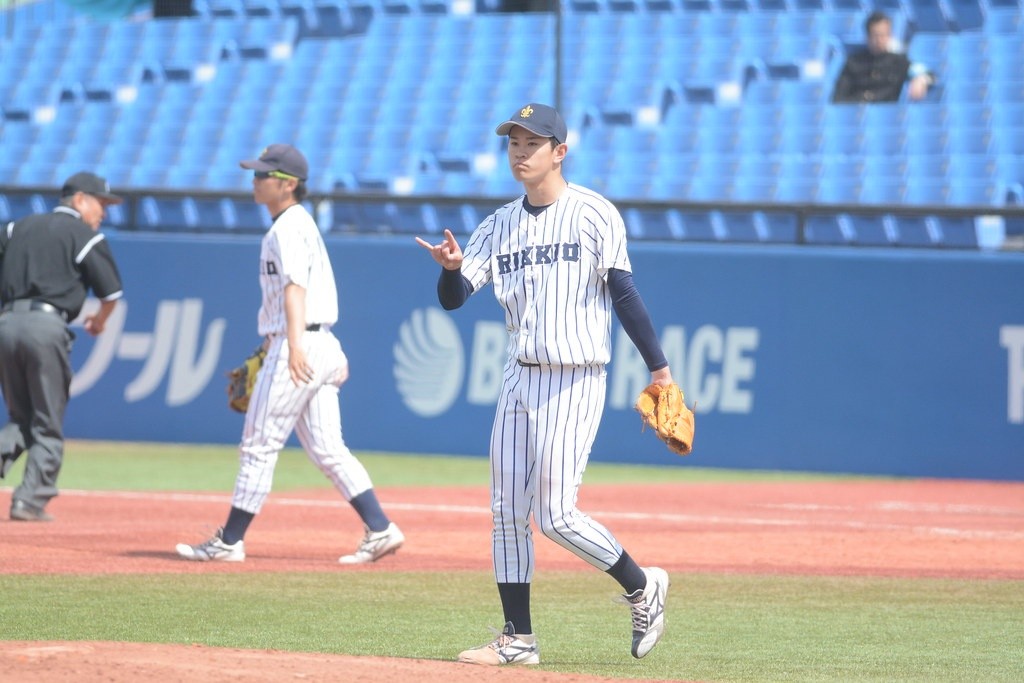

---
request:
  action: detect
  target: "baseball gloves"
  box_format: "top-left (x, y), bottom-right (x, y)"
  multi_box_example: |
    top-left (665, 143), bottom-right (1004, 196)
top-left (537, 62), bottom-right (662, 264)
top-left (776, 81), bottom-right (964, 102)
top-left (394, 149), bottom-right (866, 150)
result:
top-left (632, 382), bottom-right (698, 458)
top-left (223, 344), bottom-right (268, 414)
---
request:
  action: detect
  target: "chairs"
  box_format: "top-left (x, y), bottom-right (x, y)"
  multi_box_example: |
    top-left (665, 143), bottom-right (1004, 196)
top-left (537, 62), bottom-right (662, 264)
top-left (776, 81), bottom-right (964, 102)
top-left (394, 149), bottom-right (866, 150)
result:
top-left (1, 0), bottom-right (1023, 251)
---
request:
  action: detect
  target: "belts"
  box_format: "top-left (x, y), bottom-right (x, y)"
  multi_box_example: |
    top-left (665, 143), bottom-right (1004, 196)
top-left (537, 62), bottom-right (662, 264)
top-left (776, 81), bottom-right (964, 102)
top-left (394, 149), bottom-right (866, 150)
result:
top-left (516, 358), bottom-right (540, 367)
top-left (0, 299), bottom-right (71, 321)
top-left (307, 324), bottom-right (321, 333)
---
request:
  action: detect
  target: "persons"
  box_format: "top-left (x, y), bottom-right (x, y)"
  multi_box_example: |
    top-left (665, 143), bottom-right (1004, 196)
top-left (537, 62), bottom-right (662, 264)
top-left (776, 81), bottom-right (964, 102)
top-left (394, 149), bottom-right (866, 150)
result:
top-left (415, 103), bottom-right (685, 665)
top-left (176, 143), bottom-right (404, 564)
top-left (0, 172), bottom-right (123, 521)
top-left (828, 11), bottom-right (933, 103)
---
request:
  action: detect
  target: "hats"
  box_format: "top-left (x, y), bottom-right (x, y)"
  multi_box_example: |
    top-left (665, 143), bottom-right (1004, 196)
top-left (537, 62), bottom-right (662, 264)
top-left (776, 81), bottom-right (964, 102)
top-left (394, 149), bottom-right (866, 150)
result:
top-left (495, 104), bottom-right (567, 144)
top-left (240, 143), bottom-right (308, 181)
top-left (61, 172), bottom-right (122, 204)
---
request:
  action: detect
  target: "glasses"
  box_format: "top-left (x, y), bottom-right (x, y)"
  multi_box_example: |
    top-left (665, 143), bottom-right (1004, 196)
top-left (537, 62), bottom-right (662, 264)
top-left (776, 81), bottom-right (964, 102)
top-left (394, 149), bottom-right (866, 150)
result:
top-left (254, 170), bottom-right (299, 182)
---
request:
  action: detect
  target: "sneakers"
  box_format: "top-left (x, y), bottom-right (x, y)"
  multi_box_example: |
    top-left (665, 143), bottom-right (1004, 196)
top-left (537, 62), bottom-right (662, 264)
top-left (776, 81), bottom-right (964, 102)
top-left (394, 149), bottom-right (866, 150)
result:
top-left (611, 566), bottom-right (671, 659)
top-left (339, 522), bottom-right (405, 565)
top-left (175, 527), bottom-right (246, 562)
top-left (457, 620), bottom-right (539, 666)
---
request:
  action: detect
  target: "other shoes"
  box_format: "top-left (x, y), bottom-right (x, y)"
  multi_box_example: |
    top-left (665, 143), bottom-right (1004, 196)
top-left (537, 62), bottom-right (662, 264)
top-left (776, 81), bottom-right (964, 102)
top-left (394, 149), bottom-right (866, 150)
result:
top-left (10, 499), bottom-right (52, 521)
